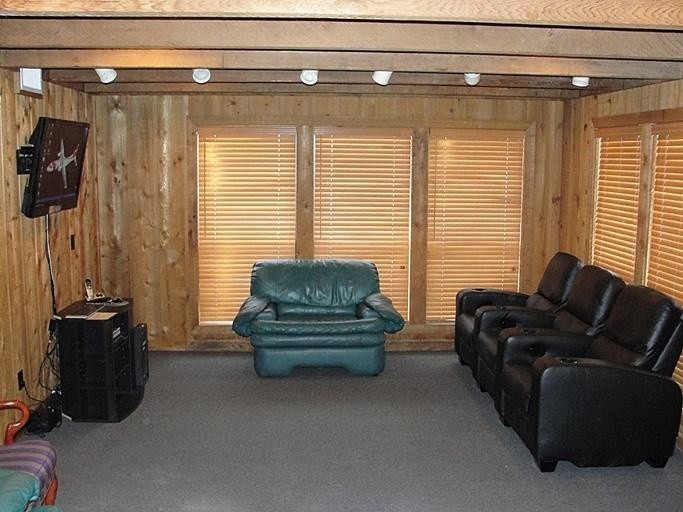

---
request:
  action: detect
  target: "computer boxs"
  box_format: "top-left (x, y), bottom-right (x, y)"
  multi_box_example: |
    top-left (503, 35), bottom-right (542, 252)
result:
top-left (133, 323), bottom-right (149, 386)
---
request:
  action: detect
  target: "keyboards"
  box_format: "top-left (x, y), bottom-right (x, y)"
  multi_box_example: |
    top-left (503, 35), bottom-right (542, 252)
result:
top-left (66, 303), bottom-right (105, 320)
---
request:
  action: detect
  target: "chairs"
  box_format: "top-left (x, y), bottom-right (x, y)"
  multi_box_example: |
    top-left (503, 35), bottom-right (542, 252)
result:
top-left (232, 258), bottom-right (404, 379)
top-left (0, 400), bottom-right (58, 512)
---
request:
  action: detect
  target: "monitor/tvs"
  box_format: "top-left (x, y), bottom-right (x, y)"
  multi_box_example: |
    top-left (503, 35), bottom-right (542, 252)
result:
top-left (22, 116), bottom-right (91, 218)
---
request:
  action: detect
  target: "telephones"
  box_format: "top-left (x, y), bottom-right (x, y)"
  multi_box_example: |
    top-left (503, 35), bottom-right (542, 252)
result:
top-left (83, 278), bottom-right (105, 302)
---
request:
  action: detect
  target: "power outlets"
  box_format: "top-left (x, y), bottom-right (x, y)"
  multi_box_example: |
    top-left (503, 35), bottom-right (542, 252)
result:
top-left (18, 370), bottom-right (25, 391)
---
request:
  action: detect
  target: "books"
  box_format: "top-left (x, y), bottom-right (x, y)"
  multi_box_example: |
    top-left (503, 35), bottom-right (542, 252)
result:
top-left (111, 325), bottom-right (121, 334)
top-left (111, 331), bottom-right (121, 339)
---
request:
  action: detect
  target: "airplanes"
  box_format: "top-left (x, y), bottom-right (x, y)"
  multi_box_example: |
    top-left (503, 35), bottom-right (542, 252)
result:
top-left (46, 137), bottom-right (80, 191)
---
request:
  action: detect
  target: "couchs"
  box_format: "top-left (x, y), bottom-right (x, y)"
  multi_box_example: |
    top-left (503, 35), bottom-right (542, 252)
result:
top-left (456, 253), bottom-right (586, 376)
top-left (494, 281), bottom-right (681, 472)
top-left (469, 262), bottom-right (627, 430)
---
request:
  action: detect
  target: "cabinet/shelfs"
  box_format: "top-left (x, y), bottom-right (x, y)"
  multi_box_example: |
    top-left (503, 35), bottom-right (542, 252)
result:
top-left (54, 296), bottom-right (150, 423)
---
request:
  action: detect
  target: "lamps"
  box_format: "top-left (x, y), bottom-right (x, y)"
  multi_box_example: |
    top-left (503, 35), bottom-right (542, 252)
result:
top-left (462, 71), bottom-right (482, 87)
top-left (94, 67), bottom-right (119, 85)
top-left (299, 69), bottom-right (320, 85)
top-left (571, 76), bottom-right (591, 88)
top-left (371, 70), bottom-right (395, 87)
top-left (191, 68), bottom-right (212, 85)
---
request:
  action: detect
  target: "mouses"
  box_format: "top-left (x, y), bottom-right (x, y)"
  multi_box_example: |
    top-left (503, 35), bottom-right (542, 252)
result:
top-left (111, 296), bottom-right (124, 303)
